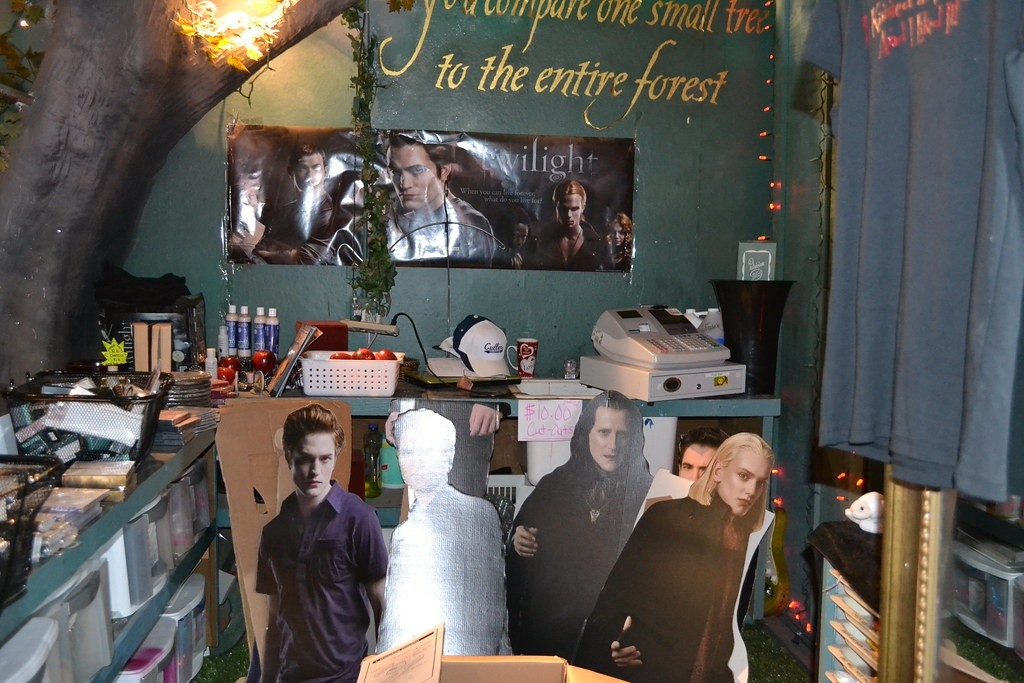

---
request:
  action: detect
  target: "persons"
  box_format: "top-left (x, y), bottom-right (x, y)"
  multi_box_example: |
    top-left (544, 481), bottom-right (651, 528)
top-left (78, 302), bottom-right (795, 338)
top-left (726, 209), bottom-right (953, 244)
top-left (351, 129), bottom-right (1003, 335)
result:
top-left (256, 399), bottom-right (774, 683)
top-left (229, 132), bottom-right (631, 270)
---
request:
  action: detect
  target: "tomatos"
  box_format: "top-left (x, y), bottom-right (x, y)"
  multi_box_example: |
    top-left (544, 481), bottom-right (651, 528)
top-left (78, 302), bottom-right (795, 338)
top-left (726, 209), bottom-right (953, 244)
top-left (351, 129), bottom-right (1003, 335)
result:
top-left (330, 349), bottom-right (397, 360)
top-left (252, 349), bottom-right (277, 372)
top-left (218, 357), bottom-right (241, 386)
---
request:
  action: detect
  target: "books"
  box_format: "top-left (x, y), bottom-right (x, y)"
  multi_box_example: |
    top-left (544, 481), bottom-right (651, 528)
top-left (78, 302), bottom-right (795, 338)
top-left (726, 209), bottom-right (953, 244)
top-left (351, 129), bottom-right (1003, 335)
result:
top-left (36, 456), bottom-right (137, 530)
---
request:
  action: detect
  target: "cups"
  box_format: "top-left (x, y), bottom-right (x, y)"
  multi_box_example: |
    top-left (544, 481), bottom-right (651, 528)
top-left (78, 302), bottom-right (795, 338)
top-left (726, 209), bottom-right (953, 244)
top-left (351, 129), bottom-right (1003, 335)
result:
top-left (506, 338), bottom-right (539, 380)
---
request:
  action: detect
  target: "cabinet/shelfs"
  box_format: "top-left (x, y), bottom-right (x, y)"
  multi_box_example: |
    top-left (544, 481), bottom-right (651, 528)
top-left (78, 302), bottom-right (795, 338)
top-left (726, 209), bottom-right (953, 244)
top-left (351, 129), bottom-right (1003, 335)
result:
top-left (0, 428), bottom-right (236, 682)
top-left (303, 391), bottom-right (779, 621)
top-left (811, 460), bottom-right (1024, 683)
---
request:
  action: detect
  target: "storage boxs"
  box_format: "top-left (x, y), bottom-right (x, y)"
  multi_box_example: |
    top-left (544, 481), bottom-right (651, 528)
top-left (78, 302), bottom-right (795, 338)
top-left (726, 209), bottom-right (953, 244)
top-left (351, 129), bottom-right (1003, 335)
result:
top-left (526, 416), bottom-right (679, 488)
top-left (356, 621), bottom-right (634, 683)
top-left (0, 457), bottom-right (210, 683)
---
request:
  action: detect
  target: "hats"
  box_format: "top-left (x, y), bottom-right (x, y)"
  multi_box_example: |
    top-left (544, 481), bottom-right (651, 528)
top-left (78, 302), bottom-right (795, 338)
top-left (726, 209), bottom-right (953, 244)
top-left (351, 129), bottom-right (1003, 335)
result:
top-left (453, 314), bottom-right (512, 377)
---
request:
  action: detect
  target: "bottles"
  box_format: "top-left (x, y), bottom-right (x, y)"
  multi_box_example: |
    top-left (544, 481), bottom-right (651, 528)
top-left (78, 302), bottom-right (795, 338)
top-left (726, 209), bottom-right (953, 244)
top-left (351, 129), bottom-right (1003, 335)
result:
top-left (217, 325), bottom-right (228, 361)
top-left (206, 348), bottom-right (218, 380)
top-left (265, 308), bottom-right (281, 357)
top-left (238, 306), bottom-right (252, 358)
top-left (225, 302), bottom-right (238, 356)
top-left (254, 307), bottom-right (267, 351)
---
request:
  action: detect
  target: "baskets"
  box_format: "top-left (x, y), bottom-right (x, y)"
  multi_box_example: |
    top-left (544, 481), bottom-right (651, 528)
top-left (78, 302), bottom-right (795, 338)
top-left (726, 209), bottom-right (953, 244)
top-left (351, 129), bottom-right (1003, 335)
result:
top-left (0, 453), bottom-right (66, 611)
top-left (0, 369), bottom-right (176, 469)
top-left (299, 350), bottom-right (405, 398)
